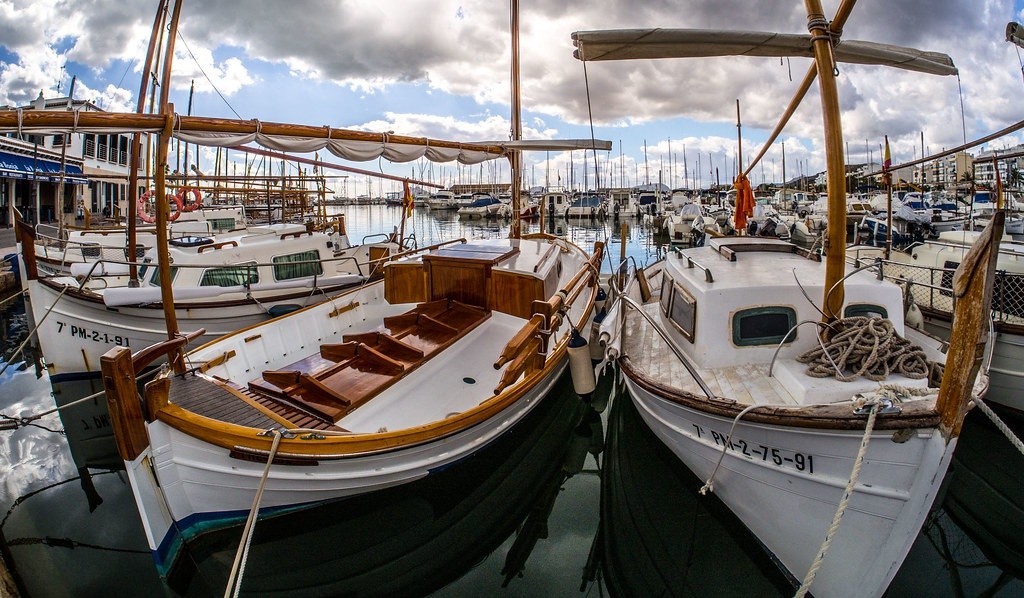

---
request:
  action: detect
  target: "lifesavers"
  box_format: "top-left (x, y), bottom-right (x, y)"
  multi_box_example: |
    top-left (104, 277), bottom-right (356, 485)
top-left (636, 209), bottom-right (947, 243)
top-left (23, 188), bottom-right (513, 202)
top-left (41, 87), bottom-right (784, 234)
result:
top-left (138, 190), bottom-right (158, 223)
top-left (989, 192), bottom-right (998, 202)
top-left (602, 204), bottom-right (608, 213)
top-left (175, 187), bottom-right (202, 213)
top-left (165, 194), bottom-right (182, 223)
top-left (732, 179), bottom-right (751, 228)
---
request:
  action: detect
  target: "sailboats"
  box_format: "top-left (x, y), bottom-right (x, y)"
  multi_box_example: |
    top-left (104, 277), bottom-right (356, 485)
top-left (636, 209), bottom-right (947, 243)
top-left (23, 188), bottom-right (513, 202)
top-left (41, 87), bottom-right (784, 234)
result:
top-left (1, 0), bottom-right (599, 583)
top-left (569, 0), bottom-right (1024, 598)
top-left (381, 136), bottom-right (1023, 244)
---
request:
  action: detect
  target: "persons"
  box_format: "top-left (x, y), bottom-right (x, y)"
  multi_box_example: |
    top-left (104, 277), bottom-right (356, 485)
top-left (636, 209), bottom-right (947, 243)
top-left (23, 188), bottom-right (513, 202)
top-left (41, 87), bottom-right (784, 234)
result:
top-left (103, 204), bottom-right (111, 218)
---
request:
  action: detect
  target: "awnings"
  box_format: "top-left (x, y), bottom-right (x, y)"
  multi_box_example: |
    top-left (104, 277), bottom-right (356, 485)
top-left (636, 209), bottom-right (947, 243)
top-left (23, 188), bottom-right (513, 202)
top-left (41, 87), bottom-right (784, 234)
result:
top-left (0, 152), bottom-right (89, 184)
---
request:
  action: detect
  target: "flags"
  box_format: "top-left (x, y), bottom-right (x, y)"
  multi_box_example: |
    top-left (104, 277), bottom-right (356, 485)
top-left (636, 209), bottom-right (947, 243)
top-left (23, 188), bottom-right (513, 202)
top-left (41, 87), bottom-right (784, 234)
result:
top-left (405, 178), bottom-right (414, 218)
top-left (883, 137), bottom-right (891, 184)
top-left (313, 153), bottom-right (319, 174)
